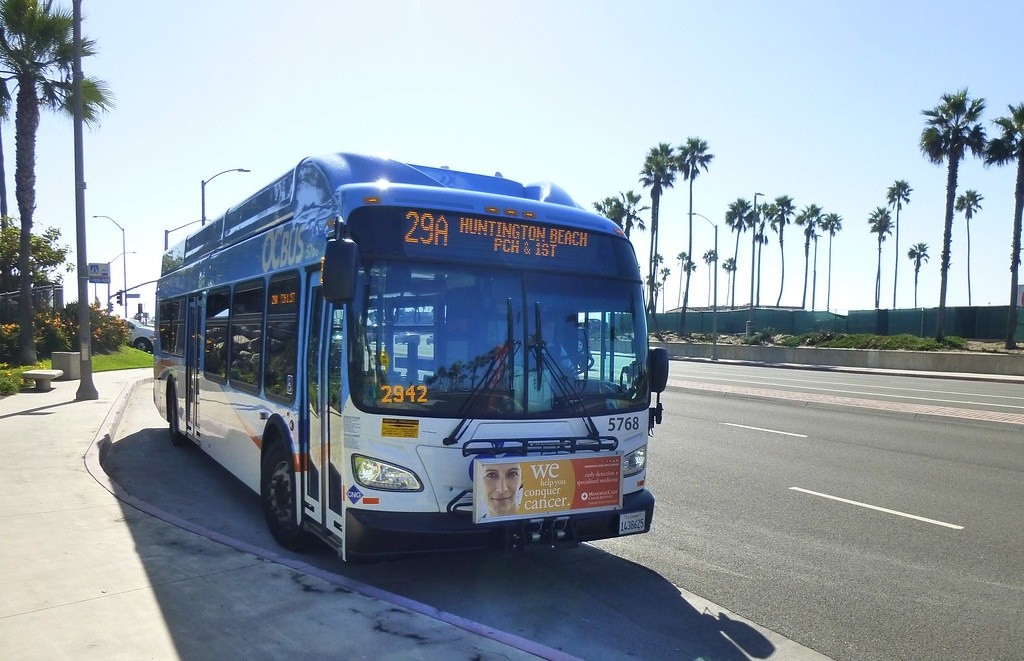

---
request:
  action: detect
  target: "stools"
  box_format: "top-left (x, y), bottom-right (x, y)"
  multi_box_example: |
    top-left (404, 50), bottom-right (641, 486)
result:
top-left (23, 370), bottom-right (64, 392)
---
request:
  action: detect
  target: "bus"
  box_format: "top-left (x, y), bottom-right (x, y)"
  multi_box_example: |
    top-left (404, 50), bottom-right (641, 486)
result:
top-left (153, 150), bottom-right (670, 563)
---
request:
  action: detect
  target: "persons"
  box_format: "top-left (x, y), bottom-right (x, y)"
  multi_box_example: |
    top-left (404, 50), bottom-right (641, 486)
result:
top-left (480, 463), bottom-right (522, 517)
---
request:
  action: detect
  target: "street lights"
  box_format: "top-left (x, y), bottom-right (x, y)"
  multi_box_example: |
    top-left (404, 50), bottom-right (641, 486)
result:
top-left (201, 168), bottom-right (251, 227)
top-left (745, 193), bottom-right (764, 337)
top-left (108, 252), bottom-right (135, 317)
top-left (688, 213), bottom-right (718, 360)
top-left (93, 215), bottom-right (127, 318)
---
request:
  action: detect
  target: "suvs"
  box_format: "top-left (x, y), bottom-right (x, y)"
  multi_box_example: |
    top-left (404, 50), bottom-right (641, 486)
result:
top-left (119, 317), bottom-right (154, 354)
